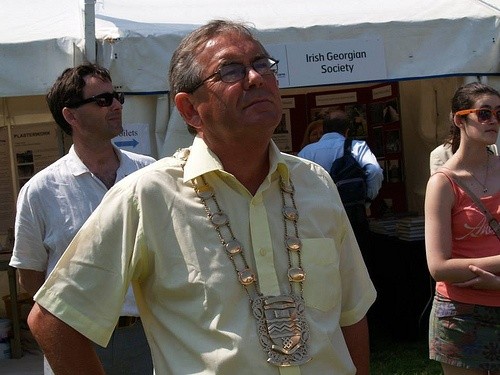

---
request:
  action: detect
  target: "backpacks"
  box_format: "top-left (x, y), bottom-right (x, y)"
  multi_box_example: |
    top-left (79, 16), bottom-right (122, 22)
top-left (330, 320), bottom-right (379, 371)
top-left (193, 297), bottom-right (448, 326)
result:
top-left (329, 139), bottom-right (368, 208)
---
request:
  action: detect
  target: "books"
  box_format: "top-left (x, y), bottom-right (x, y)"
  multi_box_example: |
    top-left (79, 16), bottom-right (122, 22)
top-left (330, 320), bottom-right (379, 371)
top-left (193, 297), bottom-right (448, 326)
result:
top-left (366, 209), bottom-right (424, 242)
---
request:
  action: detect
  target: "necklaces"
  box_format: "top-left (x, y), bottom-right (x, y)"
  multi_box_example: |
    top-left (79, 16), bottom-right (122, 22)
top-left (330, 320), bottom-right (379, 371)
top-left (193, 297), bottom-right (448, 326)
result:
top-left (174, 148), bottom-right (313, 366)
top-left (456, 154), bottom-right (491, 193)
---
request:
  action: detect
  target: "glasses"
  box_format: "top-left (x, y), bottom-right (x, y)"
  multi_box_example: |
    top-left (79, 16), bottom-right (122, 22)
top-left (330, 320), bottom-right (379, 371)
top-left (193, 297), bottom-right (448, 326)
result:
top-left (188, 57), bottom-right (279, 94)
top-left (73, 90), bottom-right (124, 108)
top-left (455, 108), bottom-right (500, 124)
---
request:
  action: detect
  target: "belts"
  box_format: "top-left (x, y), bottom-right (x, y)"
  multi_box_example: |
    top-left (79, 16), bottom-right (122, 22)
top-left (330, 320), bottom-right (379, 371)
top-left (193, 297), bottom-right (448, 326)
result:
top-left (117, 315), bottom-right (141, 328)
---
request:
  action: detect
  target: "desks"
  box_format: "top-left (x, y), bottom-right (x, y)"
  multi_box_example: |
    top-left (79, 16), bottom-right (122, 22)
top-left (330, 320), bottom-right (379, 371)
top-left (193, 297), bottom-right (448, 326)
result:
top-left (365, 232), bottom-right (427, 346)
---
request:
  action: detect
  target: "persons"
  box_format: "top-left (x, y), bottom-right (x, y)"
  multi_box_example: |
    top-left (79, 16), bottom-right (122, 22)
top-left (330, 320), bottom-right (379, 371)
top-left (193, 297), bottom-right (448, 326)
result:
top-left (300, 120), bottom-right (324, 150)
top-left (8, 65), bottom-right (156, 375)
top-left (27, 24), bottom-right (378, 375)
top-left (422, 81), bottom-right (500, 375)
top-left (297, 110), bottom-right (385, 350)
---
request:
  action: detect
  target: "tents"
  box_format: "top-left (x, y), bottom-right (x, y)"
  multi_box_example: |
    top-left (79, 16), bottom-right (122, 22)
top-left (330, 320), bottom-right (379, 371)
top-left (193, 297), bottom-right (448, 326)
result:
top-left (1, 0), bottom-right (500, 243)
